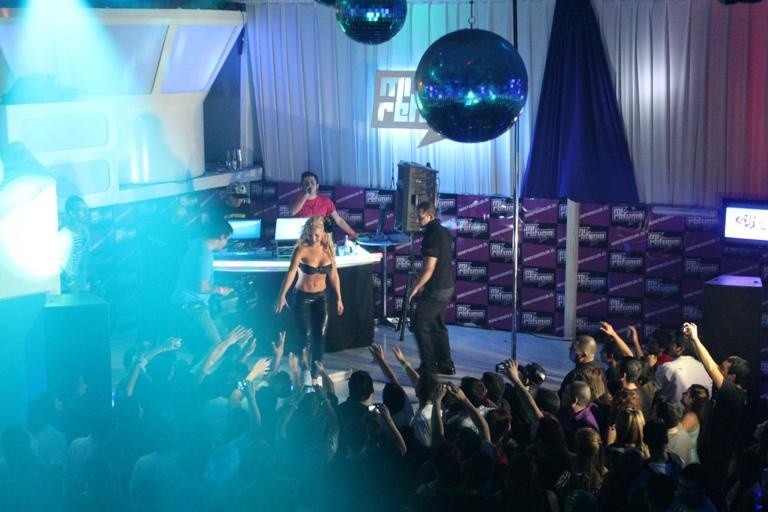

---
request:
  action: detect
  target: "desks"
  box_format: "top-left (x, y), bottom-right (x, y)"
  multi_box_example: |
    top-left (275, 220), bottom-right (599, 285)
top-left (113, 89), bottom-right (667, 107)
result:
top-left (356, 232), bottom-right (412, 329)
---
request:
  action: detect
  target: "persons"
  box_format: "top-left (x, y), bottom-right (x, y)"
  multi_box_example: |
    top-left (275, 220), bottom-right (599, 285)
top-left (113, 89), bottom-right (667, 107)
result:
top-left (173, 216), bottom-right (234, 353)
top-left (272, 215), bottom-right (344, 387)
top-left (406, 199), bottom-right (458, 376)
top-left (289, 171), bottom-right (359, 242)
top-left (60, 195), bottom-right (92, 294)
top-left (1, 322), bottom-right (768, 511)
top-left (214, 181), bottom-right (254, 249)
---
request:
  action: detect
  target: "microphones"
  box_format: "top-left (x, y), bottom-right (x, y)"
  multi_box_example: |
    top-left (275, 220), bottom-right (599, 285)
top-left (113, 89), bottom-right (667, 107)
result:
top-left (308, 187), bottom-right (312, 194)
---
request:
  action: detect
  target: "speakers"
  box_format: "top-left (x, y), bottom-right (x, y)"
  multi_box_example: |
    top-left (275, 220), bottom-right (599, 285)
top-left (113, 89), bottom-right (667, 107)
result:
top-left (44, 294), bottom-right (112, 404)
top-left (395, 162), bottom-right (439, 232)
top-left (700, 275), bottom-right (762, 364)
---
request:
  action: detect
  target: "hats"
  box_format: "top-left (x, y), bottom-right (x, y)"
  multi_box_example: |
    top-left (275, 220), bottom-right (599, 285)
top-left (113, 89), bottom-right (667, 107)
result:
top-left (518, 360), bottom-right (546, 386)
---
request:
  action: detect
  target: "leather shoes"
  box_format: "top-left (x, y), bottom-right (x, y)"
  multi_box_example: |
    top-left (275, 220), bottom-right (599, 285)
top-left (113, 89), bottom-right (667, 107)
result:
top-left (439, 367), bottom-right (456, 375)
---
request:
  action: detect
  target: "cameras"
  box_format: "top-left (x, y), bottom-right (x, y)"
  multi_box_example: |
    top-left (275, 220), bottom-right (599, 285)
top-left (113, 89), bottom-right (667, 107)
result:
top-left (368, 404), bottom-right (381, 415)
top-left (238, 379), bottom-right (247, 391)
top-left (304, 385), bottom-right (316, 394)
top-left (496, 363), bottom-right (509, 374)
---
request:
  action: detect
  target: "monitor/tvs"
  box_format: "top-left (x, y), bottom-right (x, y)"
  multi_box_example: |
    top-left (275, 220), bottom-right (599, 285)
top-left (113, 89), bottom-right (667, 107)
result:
top-left (721, 202), bottom-right (768, 247)
top-left (275, 218), bottom-right (307, 241)
top-left (226, 218), bottom-right (262, 241)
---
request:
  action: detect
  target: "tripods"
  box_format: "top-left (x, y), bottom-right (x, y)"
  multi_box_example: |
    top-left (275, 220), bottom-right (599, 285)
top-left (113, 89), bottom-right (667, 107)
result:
top-left (393, 233), bottom-right (418, 341)
